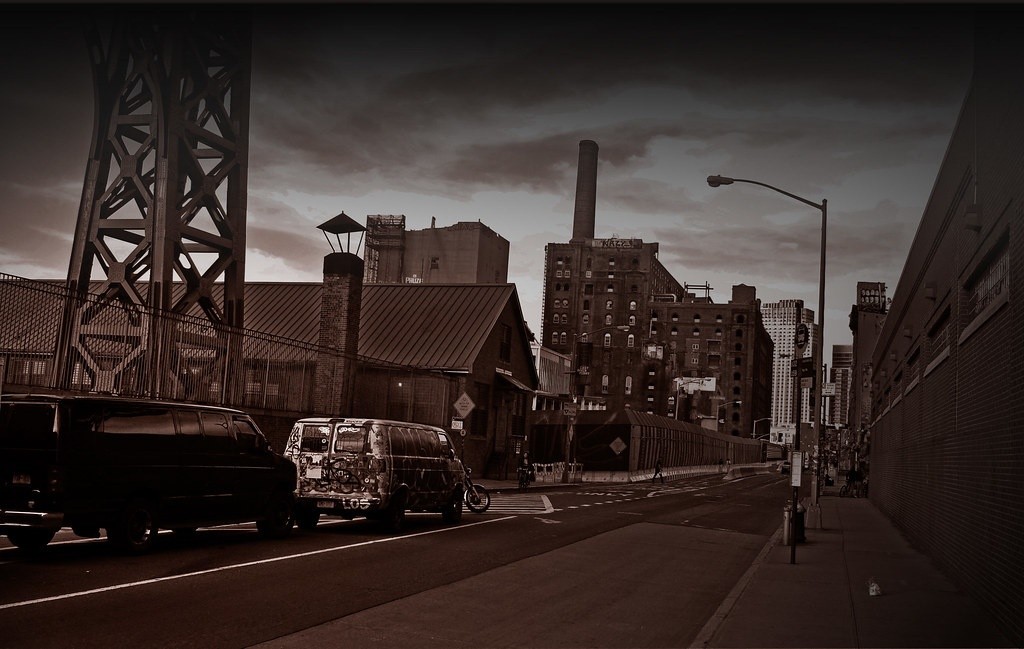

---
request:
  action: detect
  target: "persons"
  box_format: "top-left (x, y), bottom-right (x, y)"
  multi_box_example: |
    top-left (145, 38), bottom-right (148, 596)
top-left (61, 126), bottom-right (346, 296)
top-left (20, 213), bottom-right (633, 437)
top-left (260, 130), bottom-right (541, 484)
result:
top-left (824, 466), bottom-right (870, 499)
top-left (652, 457), bottom-right (665, 484)
top-left (516, 452), bottom-right (534, 484)
top-left (725, 458), bottom-right (731, 474)
top-left (718, 459), bottom-right (724, 474)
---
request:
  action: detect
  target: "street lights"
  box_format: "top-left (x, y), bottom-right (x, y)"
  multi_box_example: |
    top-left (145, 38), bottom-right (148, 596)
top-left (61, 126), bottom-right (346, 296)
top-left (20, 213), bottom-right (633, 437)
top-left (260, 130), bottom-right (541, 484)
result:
top-left (715, 401), bottom-right (743, 432)
top-left (779, 354), bottom-right (828, 478)
top-left (752, 418), bottom-right (773, 439)
top-left (674, 378), bottom-right (712, 420)
top-left (706, 174), bottom-right (829, 530)
top-left (561, 325), bottom-right (631, 483)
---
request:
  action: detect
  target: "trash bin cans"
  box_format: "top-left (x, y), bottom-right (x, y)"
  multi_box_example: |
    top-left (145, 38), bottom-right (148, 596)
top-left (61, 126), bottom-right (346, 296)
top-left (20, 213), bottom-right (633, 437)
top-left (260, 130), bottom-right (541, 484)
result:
top-left (784, 503), bottom-right (805, 543)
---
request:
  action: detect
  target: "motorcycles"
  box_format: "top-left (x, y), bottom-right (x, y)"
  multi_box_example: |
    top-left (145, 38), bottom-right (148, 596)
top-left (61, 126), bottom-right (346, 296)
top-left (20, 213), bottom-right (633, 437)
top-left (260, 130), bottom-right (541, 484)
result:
top-left (457, 451), bottom-right (491, 514)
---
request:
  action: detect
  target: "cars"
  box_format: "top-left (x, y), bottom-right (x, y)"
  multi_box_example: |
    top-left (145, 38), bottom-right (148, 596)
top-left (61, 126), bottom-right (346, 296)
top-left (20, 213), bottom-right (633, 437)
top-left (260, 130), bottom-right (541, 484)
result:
top-left (781, 461), bottom-right (791, 474)
top-left (802, 457), bottom-right (811, 470)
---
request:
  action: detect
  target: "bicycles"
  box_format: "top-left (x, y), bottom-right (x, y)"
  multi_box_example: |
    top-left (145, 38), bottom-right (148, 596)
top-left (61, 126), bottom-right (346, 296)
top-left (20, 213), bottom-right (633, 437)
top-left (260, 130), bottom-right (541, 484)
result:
top-left (516, 467), bottom-right (533, 494)
top-left (839, 472), bottom-right (866, 499)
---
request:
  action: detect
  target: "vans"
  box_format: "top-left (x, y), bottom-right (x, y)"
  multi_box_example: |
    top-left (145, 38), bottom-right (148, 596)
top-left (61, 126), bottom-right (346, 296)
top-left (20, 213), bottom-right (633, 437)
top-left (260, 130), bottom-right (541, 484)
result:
top-left (282, 418), bottom-right (465, 523)
top-left (0, 393), bottom-right (297, 550)
